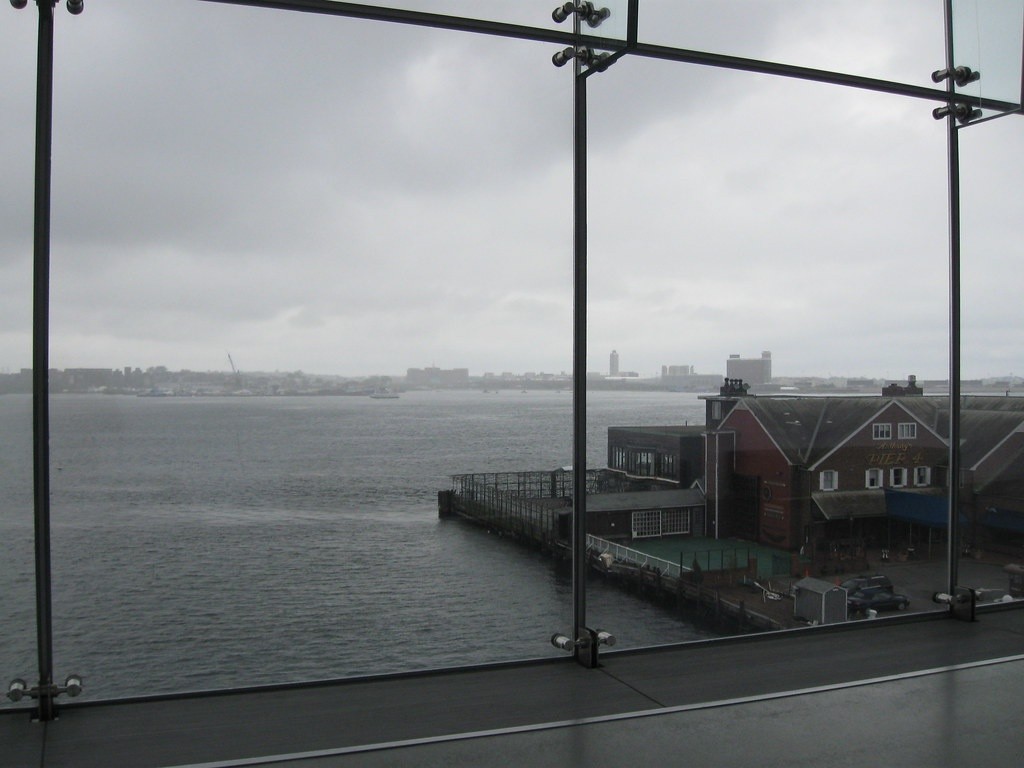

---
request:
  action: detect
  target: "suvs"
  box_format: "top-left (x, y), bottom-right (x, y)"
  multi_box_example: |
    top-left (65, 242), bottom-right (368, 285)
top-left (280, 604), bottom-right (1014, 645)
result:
top-left (839, 576), bottom-right (893, 599)
top-left (844, 591), bottom-right (911, 617)
